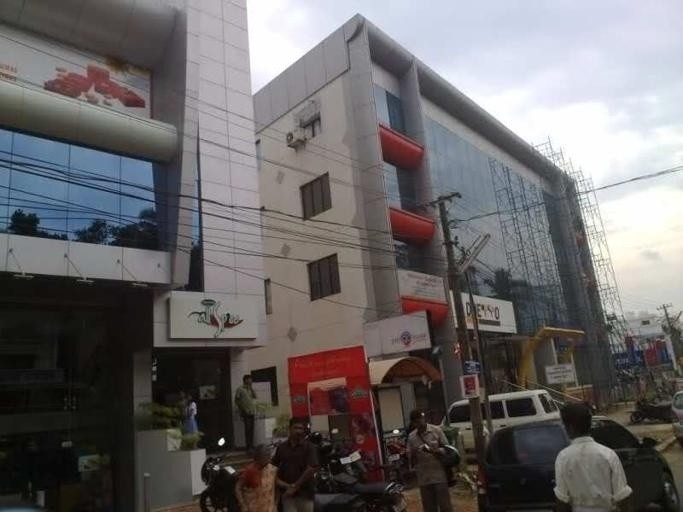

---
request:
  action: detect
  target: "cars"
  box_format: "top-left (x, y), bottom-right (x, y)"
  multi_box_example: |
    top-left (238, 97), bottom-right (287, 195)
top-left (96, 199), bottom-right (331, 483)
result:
top-left (470, 414), bottom-right (682, 512)
top-left (669, 391), bottom-right (682, 450)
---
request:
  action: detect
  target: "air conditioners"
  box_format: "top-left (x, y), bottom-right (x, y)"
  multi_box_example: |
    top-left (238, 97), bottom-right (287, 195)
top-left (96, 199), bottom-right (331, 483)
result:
top-left (286, 127), bottom-right (305, 148)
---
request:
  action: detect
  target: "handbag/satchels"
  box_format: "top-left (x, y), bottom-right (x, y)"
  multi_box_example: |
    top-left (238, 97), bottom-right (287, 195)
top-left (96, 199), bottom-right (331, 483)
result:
top-left (444, 466), bottom-right (457, 487)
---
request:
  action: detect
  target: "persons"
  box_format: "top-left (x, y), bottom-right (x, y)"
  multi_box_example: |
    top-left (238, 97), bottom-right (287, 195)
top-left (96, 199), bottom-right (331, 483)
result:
top-left (271, 417), bottom-right (322, 512)
top-left (553, 404), bottom-right (633, 512)
top-left (407, 409), bottom-right (455, 512)
top-left (235, 375), bottom-right (258, 448)
top-left (183, 392), bottom-right (198, 449)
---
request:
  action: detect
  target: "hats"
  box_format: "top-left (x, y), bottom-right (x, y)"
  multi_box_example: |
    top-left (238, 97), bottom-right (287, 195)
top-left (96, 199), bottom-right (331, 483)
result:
top-left (410, 408), bottom-right (425, 420)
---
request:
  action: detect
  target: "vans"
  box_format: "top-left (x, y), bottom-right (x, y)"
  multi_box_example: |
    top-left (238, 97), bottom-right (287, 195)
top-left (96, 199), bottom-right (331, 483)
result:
top-left (434, 386), bottom-right (571, 467)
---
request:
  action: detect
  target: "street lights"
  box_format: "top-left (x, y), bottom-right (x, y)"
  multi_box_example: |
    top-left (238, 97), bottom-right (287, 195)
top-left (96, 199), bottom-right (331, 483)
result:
top-left (447, 232), bottom-right (494, 483)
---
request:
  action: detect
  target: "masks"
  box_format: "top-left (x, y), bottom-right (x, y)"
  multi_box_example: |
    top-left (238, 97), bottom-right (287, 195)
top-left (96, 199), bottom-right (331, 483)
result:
top-left (290, 429), bottom-right (304, 447)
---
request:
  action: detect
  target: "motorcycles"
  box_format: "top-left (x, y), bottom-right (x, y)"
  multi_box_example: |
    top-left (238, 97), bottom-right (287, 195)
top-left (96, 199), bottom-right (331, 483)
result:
top-left (627, 393), bottom-right (677, 426)
top-left (198, 427), bottom-right (420, 512)
